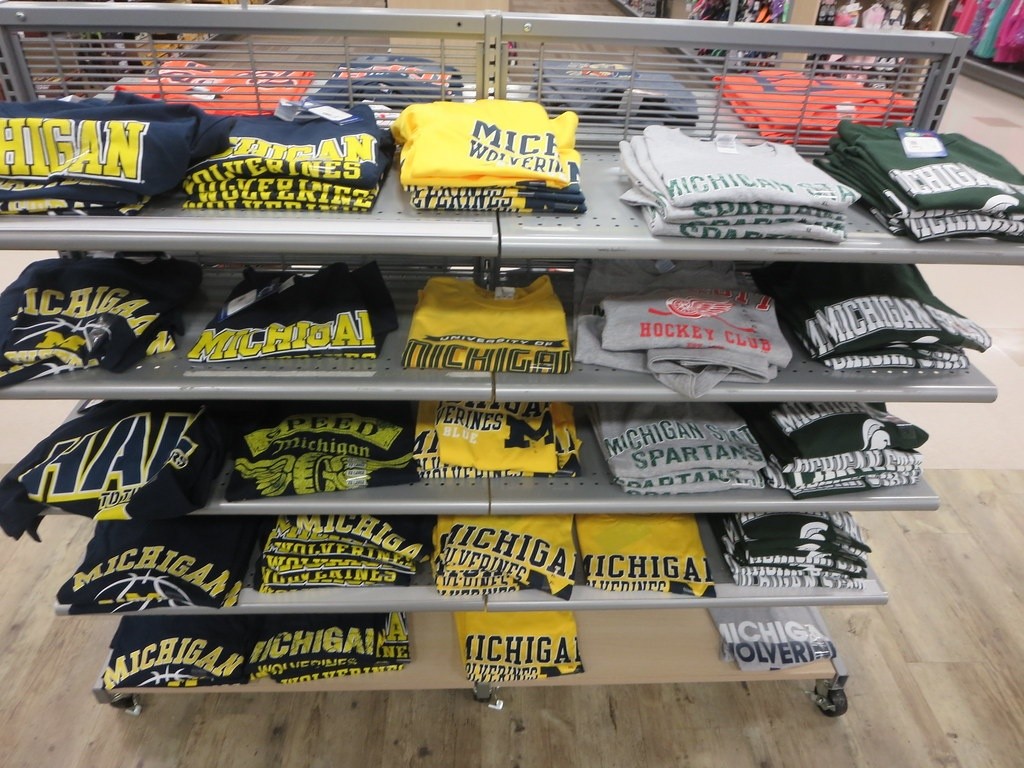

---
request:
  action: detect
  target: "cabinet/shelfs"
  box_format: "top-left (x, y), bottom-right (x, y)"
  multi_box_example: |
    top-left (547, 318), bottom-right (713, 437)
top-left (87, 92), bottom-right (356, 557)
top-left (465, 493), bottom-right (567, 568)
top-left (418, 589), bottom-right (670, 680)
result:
top-left (1, 153), bottom-right (1024, 697)
top-left (618, 0), bottom-right (951, 110)
